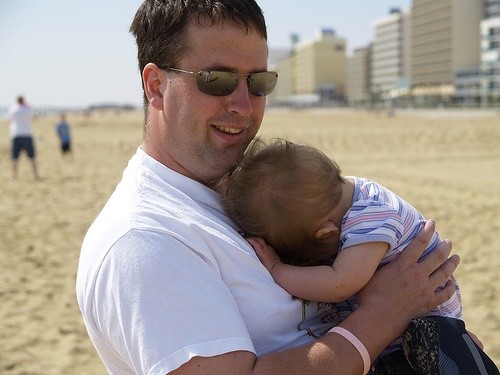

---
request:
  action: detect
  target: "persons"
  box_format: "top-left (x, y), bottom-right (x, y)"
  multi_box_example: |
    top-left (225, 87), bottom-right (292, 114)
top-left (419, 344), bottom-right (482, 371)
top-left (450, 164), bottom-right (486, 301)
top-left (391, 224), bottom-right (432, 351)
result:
top-left (76, 0), bottom-right (484, 375)
top-left (219, 134), bottom-right (462, 320)
top-left (56, 112), bottom-right (74, 161)
top-left (4, 94), bottom-right (40, 179)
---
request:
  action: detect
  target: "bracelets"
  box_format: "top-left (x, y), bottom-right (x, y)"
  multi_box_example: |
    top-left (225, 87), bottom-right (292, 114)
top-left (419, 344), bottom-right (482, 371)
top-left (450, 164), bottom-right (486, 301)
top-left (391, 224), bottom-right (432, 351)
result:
top-left (328, 327), bottom-right (371, 375)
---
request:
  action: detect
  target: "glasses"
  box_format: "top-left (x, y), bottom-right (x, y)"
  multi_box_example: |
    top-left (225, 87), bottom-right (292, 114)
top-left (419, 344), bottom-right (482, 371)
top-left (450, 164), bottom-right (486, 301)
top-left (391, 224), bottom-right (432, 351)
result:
top-left (166, 67), bottom-right (278, 96)
